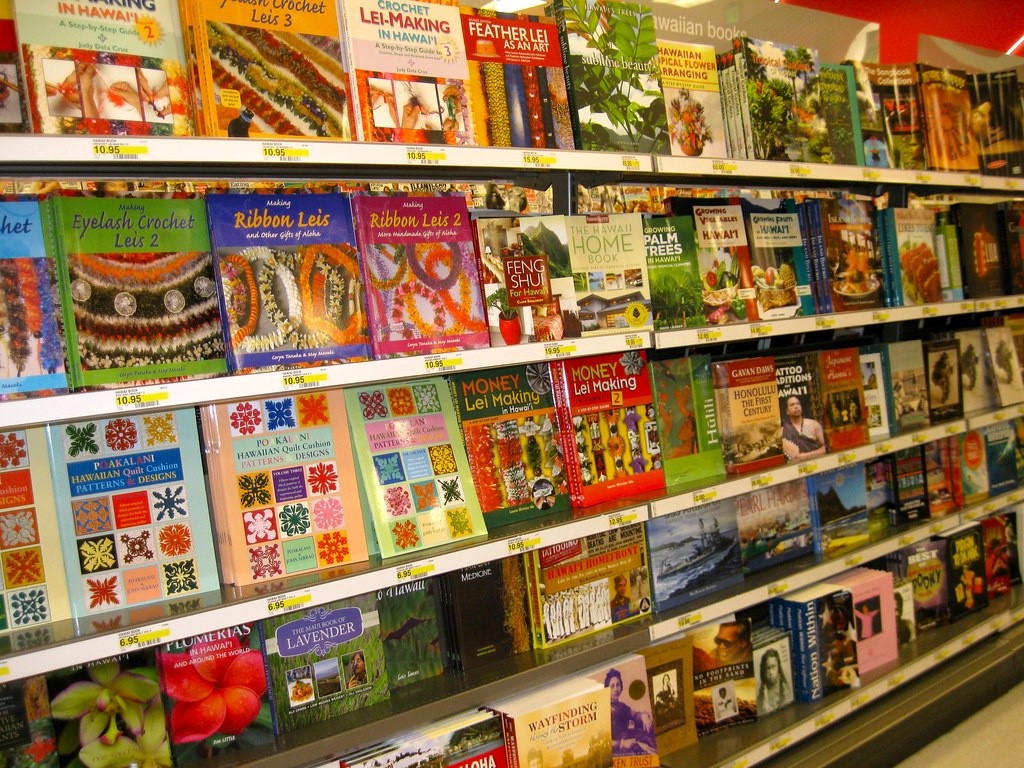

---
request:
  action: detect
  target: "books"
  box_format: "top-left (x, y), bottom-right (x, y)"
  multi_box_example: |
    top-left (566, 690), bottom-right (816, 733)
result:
top-left (1, 0), bottom-right (1024, 768)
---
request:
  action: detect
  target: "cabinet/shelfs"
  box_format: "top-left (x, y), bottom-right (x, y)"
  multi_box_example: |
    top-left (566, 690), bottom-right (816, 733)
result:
top-left (0, 135), bottom-right (1024, 768)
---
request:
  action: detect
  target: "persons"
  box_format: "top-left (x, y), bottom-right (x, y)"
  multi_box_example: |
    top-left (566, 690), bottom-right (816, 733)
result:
top-left (1005, 518), bottom-right (1020, 579)
top-left (853, 606), bottom-right (882, 639)
top-left (655, 674), bottom-right (676, 725)
top-left (604, 669), bottom-right (657, 756)
top-left (893, 369), bottom-right (930, 426)
top-left (756, 648), bottom-right (793, 718)
top-left (716, 687), bottom-right (735, 718)
top-left (487, 184), bottom-right (505, 210)
top-left (527, 747), bottom-right (543, 768)
top-left (961, 345), bottom-right (982, 390)
top-left (832, 399), bottom-right (857, 427)
top-left (984, 538), bottom-right (1009, 588)
top-left (709, 619), bottom-right (752, 666)
top-left (932, 352), bottom-right (955, 404)
top-left (781, 395), bottom-right (826, 464)
top-left (971, 101), bottom-right (1022, 151)
top-left (894, 592), bottom-right (916, 645)
top-left (610, 575), bottom-right (639, 623)
top-left (995, 340), bottom-right (1014, 386)
top-left (346, 652), bottom-right (368, 689)
